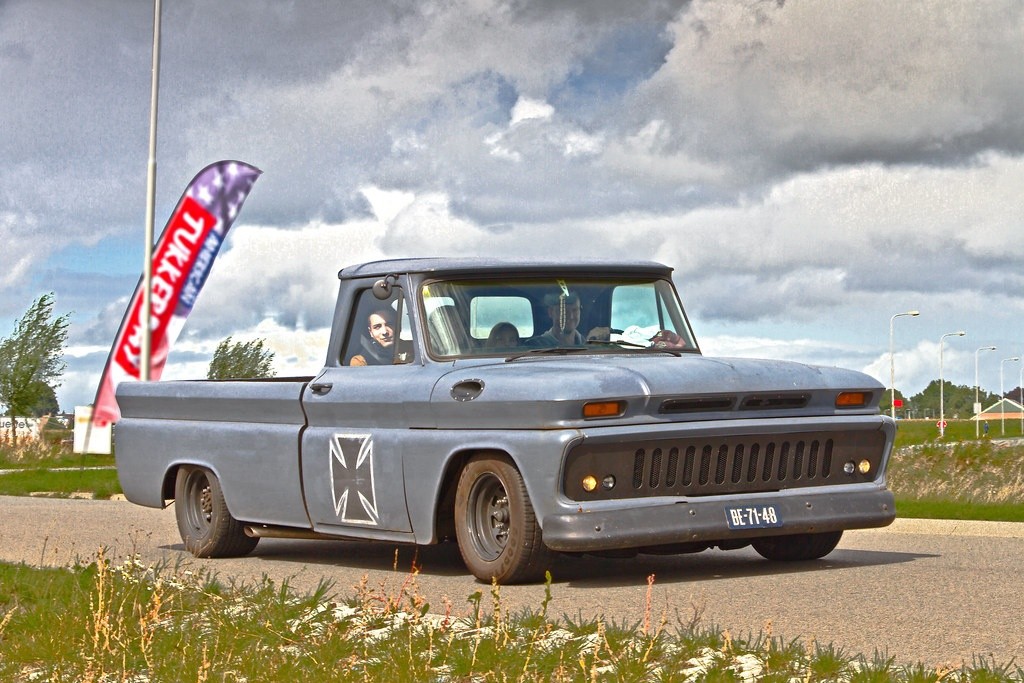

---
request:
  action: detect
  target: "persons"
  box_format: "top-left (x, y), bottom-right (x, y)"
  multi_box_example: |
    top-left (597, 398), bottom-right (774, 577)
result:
top-left (350, 307), bottom-right (413, 366)
top-left (428, 305), bottom-right (476, 352)
top-left (487, 321), bottom-right (518, 346)
top-left (523, 292), bottom-right (610, 346)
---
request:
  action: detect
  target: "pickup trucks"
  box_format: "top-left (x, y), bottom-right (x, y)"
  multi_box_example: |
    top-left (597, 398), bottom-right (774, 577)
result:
top-left (112, 255), bottom-right (896, 585)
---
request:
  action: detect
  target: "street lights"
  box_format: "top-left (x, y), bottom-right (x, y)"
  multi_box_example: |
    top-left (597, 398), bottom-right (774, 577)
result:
top-left (1000, 357), bottom-right (1020, 436)
top-left (889, 311), bottom-right (920, 420)
top-left (939, 330), bottom-right (966, 436)
top-left (974, 347), bottom-right (996, 439)
